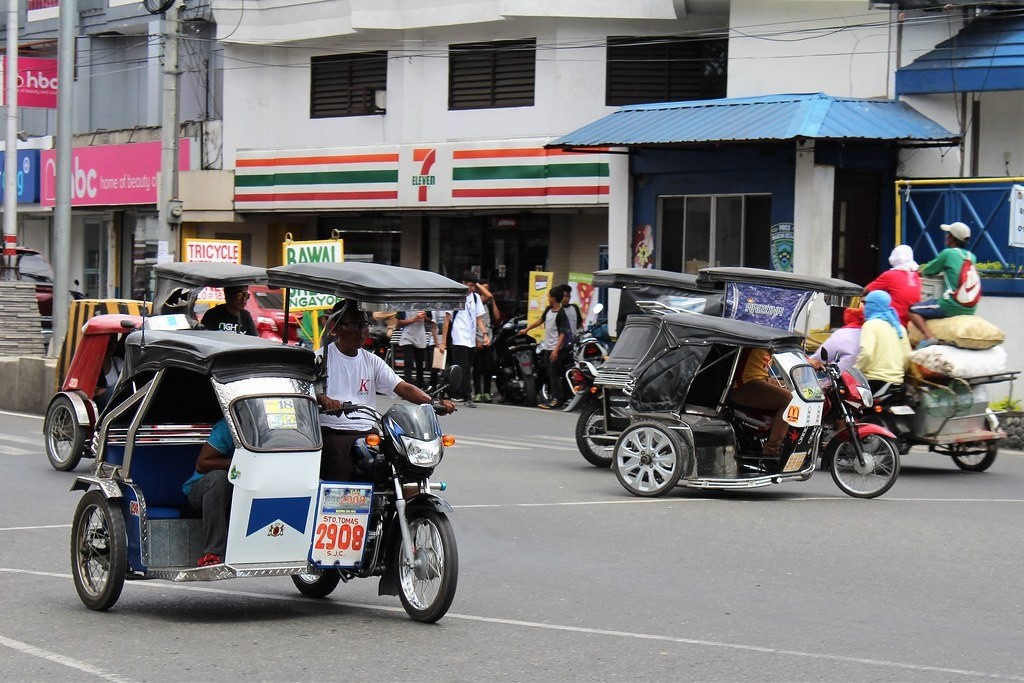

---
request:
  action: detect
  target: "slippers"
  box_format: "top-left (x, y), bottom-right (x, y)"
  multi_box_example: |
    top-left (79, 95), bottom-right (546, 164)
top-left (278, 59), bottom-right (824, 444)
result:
top-left (914, 339), bottom-right (938, 351)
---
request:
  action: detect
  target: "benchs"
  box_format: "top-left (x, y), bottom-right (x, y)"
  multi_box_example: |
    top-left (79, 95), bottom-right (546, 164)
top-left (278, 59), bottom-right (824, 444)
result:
top-left (682, 405), bottom-right (732, 437)
top-left (106, 443), bottom-right (206, 519)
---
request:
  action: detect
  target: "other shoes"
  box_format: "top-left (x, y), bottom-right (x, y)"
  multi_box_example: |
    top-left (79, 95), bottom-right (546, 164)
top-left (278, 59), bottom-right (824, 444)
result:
top-left (198, 553), bottom-right (220, 566)
top-left (760, 459), bottom-right (785, 474)
top-left (482, 393), bottom-right (492, 401)
top-left (474, 394), bottom-right (482, 401)
top-left (451, 396), bottom-right (465, 402)
top-left (538, 398), bottom-right (563, 409)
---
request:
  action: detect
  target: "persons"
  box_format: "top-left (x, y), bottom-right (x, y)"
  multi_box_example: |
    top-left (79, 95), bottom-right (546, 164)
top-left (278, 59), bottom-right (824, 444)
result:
top-left (866, 245), bottom-right (921, 326)
top-left (472, 278), bottom-right (501, 403)
top-left (811, 307), bottom-right (864, 378)
top-left (908, 223), bottom-right (982, 349)
top-left (303, 310), bottom-right (335, 350)
top-left (396, 311), bottom-right (433, 386)
top-left (428, 311), bottom-right (446, 386)
top-left (853, 290), bottom-right (912, 406)
top-left (103, 333), bottom-right (128, 387)
top-left (440, 272), bottom-right (489, 409)
top-left (201, 286), bottom-right (258, 335)
top-left (316, 300), bottom-right (455, 499)
top-left (372, 312), bottom-right (398, 338)
top-left (739, 348), bottom-right (827, 457)
top-left (182, 418), bottom-right (235, 566)
top-left (517, 284), bottom-right (585, 412)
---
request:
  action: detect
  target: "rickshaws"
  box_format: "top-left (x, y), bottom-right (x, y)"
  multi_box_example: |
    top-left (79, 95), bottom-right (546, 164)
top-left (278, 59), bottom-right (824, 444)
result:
top-left (607, 312), bottom-right (901, 499)
top-left (575, 265), bottom-right (1022, 472)
top-left (69, 262), bottom-right (471, 624)
top-left (42, 262), bottom-right (307, 471)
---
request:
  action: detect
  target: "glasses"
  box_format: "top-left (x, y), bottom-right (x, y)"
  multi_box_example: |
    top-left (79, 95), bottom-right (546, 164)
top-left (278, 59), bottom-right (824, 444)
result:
top-left (338, 321), bottom-right (372, 330)
top-left (235, 293), bottom-right (248, 299)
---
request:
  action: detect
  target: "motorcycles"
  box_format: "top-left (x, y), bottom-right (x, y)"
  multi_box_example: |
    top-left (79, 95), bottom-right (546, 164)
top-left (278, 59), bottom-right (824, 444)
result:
top-left (485, 313), bottom-right (541, 408)
top-left (559, 302), bottom-right (614, 383)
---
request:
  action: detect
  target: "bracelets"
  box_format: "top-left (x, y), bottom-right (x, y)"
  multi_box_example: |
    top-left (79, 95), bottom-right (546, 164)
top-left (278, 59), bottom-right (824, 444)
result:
top-left (483, 333), bottom-right (488, 336)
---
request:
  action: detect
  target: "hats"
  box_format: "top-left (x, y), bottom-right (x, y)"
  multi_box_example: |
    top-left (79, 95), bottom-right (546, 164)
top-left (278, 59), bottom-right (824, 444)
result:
top-left (940, 222), bottom-right (971, 243)
